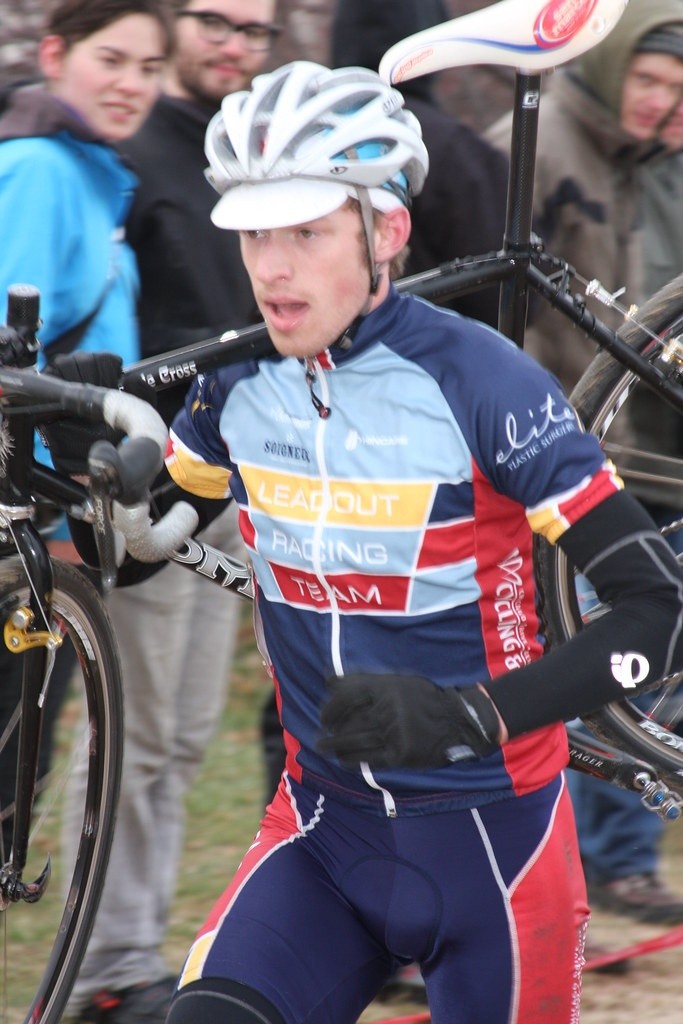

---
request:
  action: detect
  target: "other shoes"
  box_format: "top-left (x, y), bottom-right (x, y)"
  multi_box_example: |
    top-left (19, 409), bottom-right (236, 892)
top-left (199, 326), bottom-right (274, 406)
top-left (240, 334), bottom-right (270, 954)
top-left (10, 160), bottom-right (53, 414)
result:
top-left (587, 864), bottom-right (683, 924)
top-left (73, 968), bottom-right (181, 1024)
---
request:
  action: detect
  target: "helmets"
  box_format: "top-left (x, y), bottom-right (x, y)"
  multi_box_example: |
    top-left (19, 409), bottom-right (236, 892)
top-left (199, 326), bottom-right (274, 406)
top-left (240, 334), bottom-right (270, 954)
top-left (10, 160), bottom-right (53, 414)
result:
top-left (202, 58), bottom-right (431, 230)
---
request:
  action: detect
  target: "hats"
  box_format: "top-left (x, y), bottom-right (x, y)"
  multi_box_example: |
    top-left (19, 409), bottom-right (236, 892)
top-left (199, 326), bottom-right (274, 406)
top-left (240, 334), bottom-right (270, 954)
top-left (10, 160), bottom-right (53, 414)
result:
top-left (629, 20), bottom-right (683, 62)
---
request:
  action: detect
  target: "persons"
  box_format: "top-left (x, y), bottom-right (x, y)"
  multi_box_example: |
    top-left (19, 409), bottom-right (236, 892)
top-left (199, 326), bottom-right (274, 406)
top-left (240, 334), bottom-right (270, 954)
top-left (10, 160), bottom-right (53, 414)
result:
top-left (0, 0), bottom-right (175, 782)
top-left (47, 64), bottom-right (683, 1024)
top-left (44, 0), bottom-right (333, 1024)
top-left (480, 1), bottom-right (683, 922)
top-left (261, 0), bottom-right (523, 831)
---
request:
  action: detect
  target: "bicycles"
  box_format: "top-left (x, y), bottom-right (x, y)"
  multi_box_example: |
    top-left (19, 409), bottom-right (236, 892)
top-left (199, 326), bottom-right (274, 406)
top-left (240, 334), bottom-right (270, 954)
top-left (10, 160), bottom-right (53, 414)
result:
top-left (0, 0), bottom-right (683, 1024)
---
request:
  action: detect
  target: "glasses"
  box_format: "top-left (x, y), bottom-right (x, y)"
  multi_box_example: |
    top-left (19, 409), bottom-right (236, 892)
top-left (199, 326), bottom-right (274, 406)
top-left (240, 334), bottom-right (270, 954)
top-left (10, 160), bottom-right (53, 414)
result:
top-left (176, 7), bottom-right (284, 52)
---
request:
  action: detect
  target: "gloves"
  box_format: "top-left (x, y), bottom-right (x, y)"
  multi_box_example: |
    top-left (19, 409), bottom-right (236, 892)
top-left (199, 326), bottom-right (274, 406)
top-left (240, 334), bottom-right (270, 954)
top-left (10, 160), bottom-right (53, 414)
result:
top-left (316, 670), bottom-right (502, 771)
top-left (35, 350), bottom-right (159, 480)
